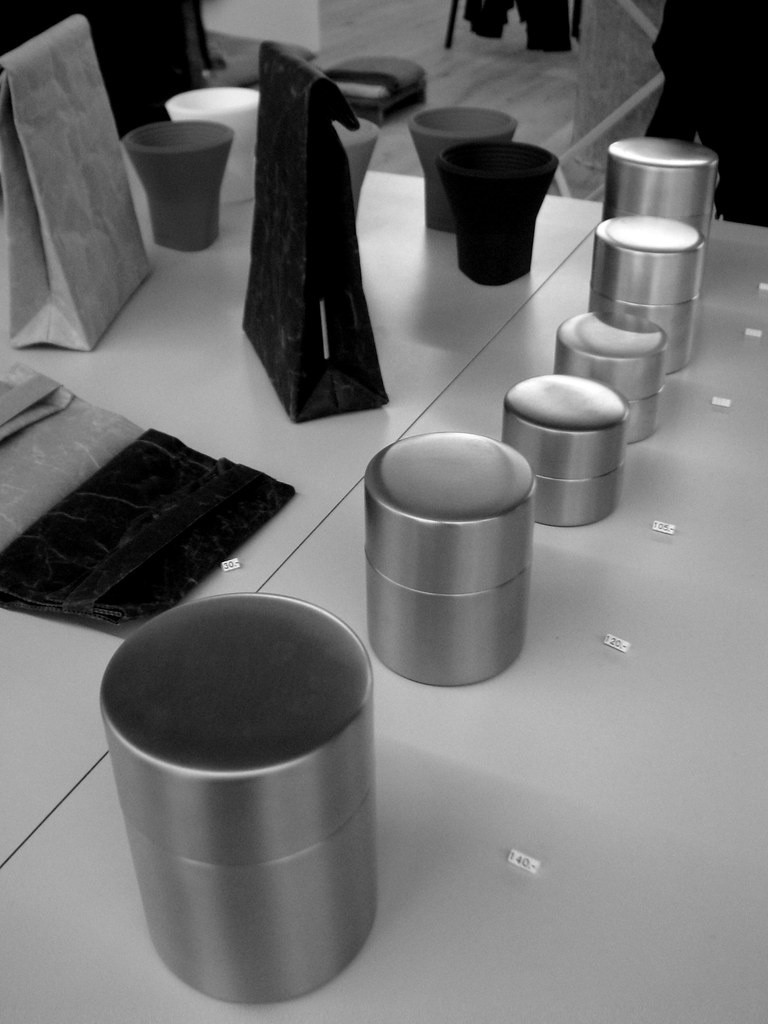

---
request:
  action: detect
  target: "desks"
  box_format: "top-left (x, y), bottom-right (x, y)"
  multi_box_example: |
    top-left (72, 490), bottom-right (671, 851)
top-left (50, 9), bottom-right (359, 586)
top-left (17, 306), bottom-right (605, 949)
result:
top-left (0, 139), bottom-right (767, 1024)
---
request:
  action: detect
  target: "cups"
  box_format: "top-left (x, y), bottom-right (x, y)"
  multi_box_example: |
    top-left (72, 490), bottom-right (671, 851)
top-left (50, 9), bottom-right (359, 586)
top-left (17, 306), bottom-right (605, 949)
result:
top-left (124, 120), bottom-right (236, 252)
top-left (331, 116), bottom-right (379, 225)
top-left (410, 103), bottom-right (519, 235)
top-left (165, 86), bottom-right (263, 201)
top-left (437, 140), bottom-right (560, 287)
